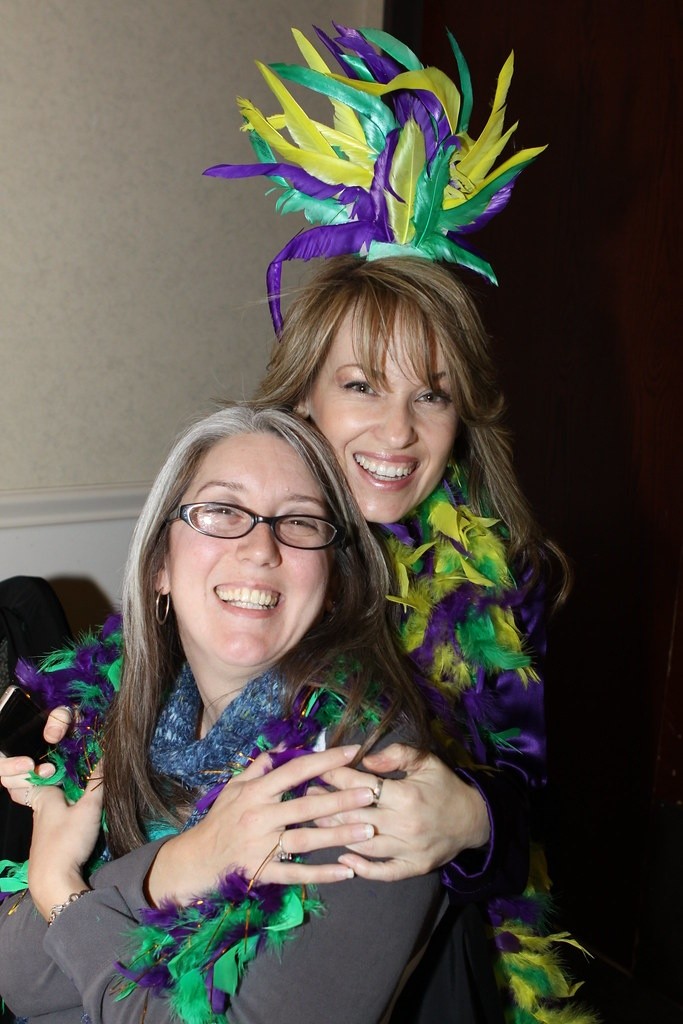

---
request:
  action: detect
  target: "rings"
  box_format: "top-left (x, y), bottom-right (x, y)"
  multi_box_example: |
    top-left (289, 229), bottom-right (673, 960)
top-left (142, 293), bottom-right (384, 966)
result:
top-left (277, 832), bottom-right (292, 860)
top-left (25, 785), bottom-right (38, 808)
top-left (370, 777), bottom-right (383, 807)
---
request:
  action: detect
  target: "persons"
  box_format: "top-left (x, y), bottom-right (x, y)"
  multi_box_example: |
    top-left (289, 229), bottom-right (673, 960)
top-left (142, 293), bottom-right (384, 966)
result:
top-left (0, 404), bottom-right (474, 1024)
top-left (0, 254), bottom-right (587, 1024)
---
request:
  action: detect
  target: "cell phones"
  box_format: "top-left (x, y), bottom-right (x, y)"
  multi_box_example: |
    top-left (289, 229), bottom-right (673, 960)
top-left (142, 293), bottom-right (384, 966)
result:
top-left (0, 684), bottom-right (62, 770)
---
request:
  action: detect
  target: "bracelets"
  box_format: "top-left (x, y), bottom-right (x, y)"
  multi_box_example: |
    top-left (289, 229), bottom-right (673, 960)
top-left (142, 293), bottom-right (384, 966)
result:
top-left (47, 890), bottom-right (91, 928)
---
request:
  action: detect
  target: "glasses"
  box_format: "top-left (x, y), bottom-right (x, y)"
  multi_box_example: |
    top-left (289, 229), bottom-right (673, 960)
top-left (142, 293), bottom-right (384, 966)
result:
top-left (166, 501), bottom-right (347, 550)
